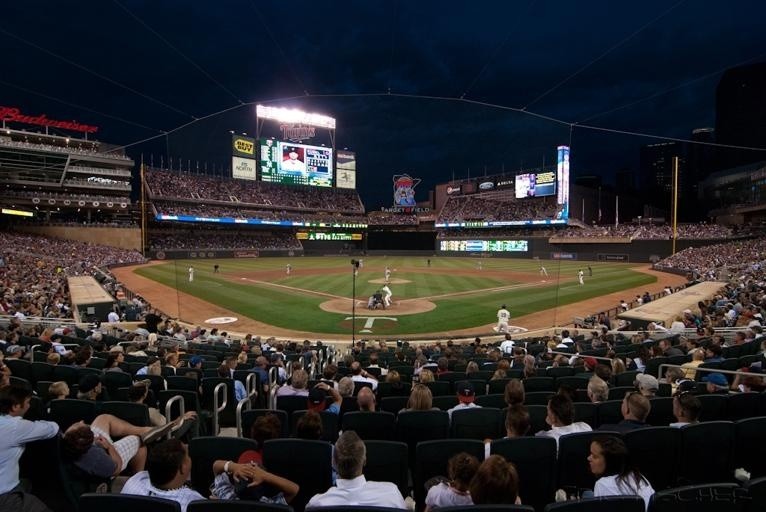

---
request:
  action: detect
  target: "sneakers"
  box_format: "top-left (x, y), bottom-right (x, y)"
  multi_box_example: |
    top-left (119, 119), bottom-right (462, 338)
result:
top-left (142, 422), bottom-right (174, 444)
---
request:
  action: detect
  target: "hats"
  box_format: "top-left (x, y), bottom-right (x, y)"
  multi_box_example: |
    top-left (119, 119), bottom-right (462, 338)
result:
top-left (50, 334), bottom-right (63, 340)
top-left (583, 357), bottom-right (597, 369)
top-left (234, 451), bottom-right (265, 500)
top-left (6, 345), bottom-right (26, 353)
top-left (190, 357), bottom-right (205, 367)
top-left (63, 328), bottom-right (72, 334)
top-left (636, 373), bottom-right (728, 394)
top-left (457, 382), bottom-right (474, 403)
top-left (146, 357), bottom-right (160, 365)
top-left (308, 387), bottom-right (326, 413)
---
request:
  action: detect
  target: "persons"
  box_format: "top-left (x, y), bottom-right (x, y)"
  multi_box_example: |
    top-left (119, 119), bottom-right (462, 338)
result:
top-left (1, 141), bottom-right (766, 511)
